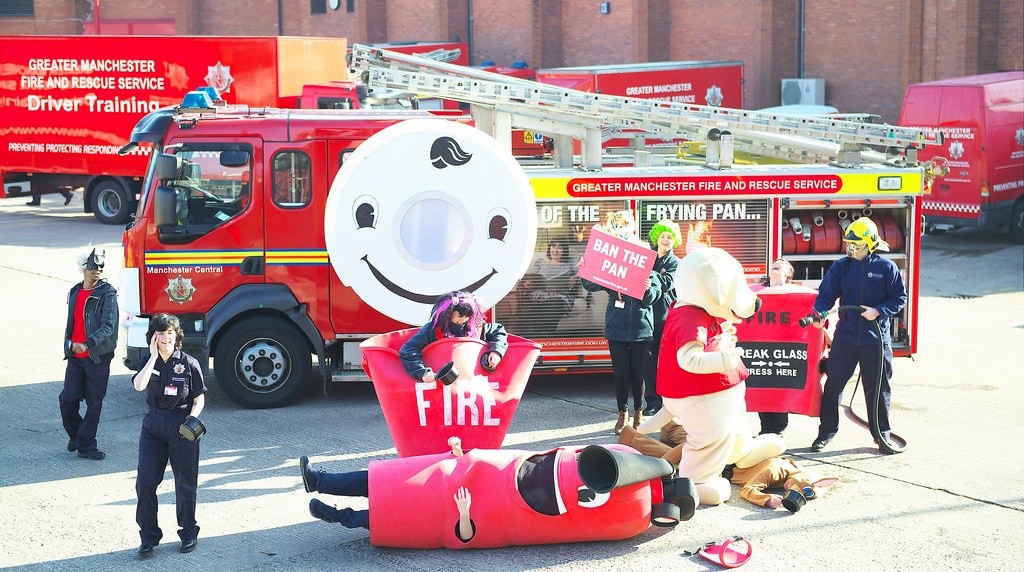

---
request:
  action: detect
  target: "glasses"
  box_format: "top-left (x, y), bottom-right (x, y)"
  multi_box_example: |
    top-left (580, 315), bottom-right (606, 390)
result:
top-left (846, 243), bottom-right (867, 252)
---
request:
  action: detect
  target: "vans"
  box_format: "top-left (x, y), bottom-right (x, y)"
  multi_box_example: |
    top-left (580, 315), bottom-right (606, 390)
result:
top-left (898, 71), bottom-right (1023, 246)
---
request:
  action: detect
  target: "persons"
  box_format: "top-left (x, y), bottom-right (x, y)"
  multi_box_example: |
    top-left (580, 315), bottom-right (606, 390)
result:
top-left (58, 249), bottom-right (119, 460)
top-left (745, 258), bottom-right (833, 439)
top-left (399, 291), bottom-right (508, 383)
top-left (577, 211), bottom-right (662, 435)
top-left (27, 175), bottom-right (73, 206)
top-left (517, 236), bottom-right (580, 334)
top-left (798, 217), bottom-right (907, 454)
top-left (300, 437), bottom-right (696, 547)
top-left (641, 219), bottom-right (684, 416)
top-left (131, 313), bottom-right (208, 554)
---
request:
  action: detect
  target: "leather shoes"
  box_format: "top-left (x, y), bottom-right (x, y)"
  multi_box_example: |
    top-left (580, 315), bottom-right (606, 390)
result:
top-left (137, 533), bottom-right (163, 557)
top-left (180, 537), bottom-right (198, 553)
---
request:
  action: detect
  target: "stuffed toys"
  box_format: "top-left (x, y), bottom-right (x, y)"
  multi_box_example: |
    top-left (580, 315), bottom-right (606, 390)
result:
top-left (655, 246), bottom-right (787, 506)
top-left (617, 418), bottom-right (839, 509)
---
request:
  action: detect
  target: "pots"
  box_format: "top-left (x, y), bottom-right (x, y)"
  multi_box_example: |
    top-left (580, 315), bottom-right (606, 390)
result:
top-left (780, 488), bottom-right (806, 512)
top-left (434, 360), bottom-right (460, 385)
top-left (178, 415), bottom-right (206, 442)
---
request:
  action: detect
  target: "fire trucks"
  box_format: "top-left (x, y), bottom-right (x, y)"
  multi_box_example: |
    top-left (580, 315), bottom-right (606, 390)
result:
top-left (0, 35), bottom-right (745, 226)
top-left (118, 44), bottom-right (951, 410)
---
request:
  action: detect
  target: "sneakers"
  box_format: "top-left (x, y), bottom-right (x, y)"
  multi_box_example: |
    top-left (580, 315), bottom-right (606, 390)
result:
top-left (300, 456), bottom-right (326, 493)
top-left (309, 498), bottom-right (337, 523)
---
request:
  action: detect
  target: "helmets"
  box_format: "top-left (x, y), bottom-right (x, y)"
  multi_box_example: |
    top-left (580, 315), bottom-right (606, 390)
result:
top-left (843, 217), bottom-right (881, 253)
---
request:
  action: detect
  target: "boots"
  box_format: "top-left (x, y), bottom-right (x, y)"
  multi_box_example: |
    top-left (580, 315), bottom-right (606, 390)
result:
top-left (614, 410), bottom-right (630, 435)
top-left (633, 410), bottom-right (642, 430)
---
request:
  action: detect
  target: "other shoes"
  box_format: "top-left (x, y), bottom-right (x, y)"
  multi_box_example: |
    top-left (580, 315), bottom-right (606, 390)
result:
top-left (68, 440), bottom-right (76, 452)
top-left (26, 201), bottom-right (40, 206)
top-left (77, 449), bottom-right (106, 460)
top-left (643, 406), bottom-right (661, 416)
top-left (758, 431), bottom-right (782, 437)
top-left (873, 438), bottom-right (901, 455)
top-left (812, 434), bottom-right (833, 452)
top-left (64, 191), bottom-right (74, 205)
